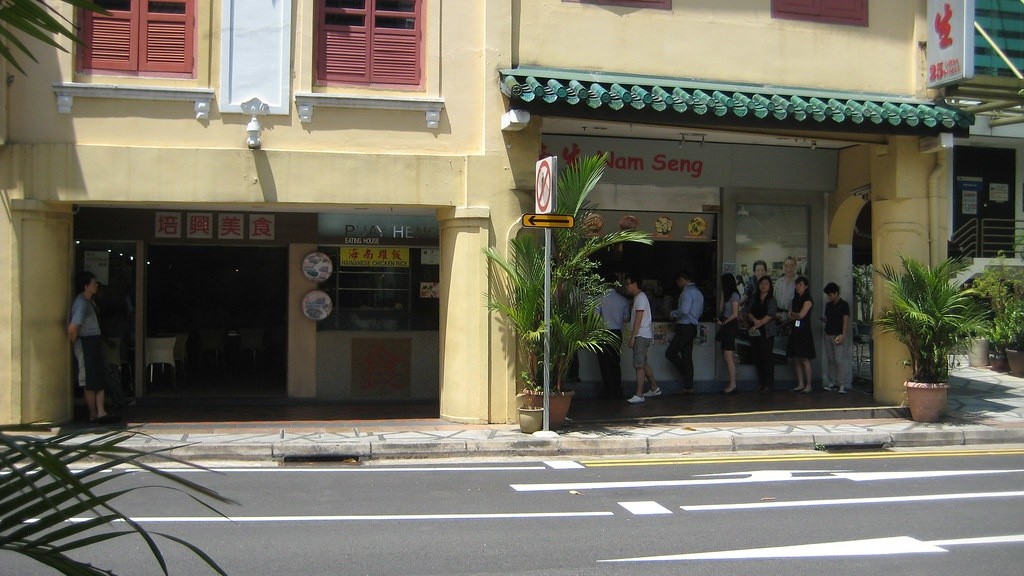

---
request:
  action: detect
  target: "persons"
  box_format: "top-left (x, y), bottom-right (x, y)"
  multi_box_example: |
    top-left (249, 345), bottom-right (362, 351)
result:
top-left (747, 260), bottom-right (767, 288)
top-left (821, 283), bottom-right (852, 394)
top-left (773, 257), bottom-right (799, 337)
top-left (736, 264), bottom-right (751, 294)
top-left (97, 335), bottom-right (134, 407)
top-left (594, 274), bottom-right (630, 397)
top-left (746, 276), bottom-right (777, 393)
top-left (785, 277), bottom-right (816, 393)
top-left (664, 272), bottom-right (704, 393)
top-left (67, 270), bottom-right (122, 424)
top-left (625, 273), bottom-right (662, 403)
top-left (716, 273), bottom-right (740, 394)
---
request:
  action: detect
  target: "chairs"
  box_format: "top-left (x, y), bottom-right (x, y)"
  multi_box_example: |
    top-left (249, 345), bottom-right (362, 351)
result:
top-left (99, 329), bottom-right (266, 383)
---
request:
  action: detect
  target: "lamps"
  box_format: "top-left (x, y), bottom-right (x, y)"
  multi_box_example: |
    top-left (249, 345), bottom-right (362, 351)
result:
top-left (247, 117), bottom-right (262, 148)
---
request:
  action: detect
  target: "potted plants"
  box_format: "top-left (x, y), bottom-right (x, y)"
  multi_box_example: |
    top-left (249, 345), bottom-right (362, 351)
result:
top-left (875, 255), bottom-right (1024, 423)
top-left (850, 262), bottom-right (874, 338)
top-left (484, 151), bottom-right (654, 433)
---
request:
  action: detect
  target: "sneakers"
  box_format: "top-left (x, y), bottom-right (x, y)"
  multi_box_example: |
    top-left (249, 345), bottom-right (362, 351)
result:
top-left (838, 385), bottom-right (847, 394)
top-left (626, 395), bottom-right (645, 403)
top-left (824, 384), bottom-right (839, 390)
top-left (643, 387), bottom-right (661, 397)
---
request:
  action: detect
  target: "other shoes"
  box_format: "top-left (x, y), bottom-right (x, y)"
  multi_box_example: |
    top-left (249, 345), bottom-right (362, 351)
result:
top-left (759, 384), bottom-right (778, 394)
top-left (671, 384), bottom-right (694, 394)
top-left (720, 386), bottom-right (737, 394)
top-left (789, 386), bottom-right (804, 392)
top-left (95, 414), bottom-right (122, 425)
top-left (801, 388), bottom-right (813, 394)
